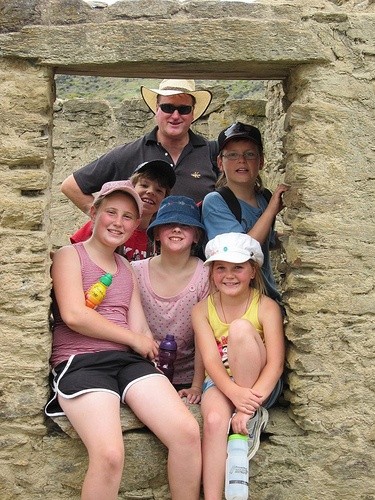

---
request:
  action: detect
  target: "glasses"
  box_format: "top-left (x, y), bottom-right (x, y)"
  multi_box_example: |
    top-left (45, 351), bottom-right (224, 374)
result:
top-left (159, 103), bottom-right (194, 115)
top-left (220, 150), bottom-right (260, 160)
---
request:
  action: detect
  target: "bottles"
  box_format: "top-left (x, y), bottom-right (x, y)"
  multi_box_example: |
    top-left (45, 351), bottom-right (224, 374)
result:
top-left (224, 434), bottom-right (249, 500)
top-left (85, 273), bottom-right (113, 309)
top-left (160, 333), bottom-right (178, 383)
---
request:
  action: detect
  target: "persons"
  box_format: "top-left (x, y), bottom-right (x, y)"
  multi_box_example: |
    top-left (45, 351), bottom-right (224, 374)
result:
top-left (192, 231), bottom-right (285, 500)
top-left (50, 179), bottom-right (204, 500)
top-left (130, 195), bottom-right (218, 405)
top-left (61, 78), bottom-right (222, 219)
top-left (49, 122), bottom-right (288, 300)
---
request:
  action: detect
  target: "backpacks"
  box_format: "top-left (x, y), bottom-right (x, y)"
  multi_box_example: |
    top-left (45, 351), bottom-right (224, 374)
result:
top-left (191, 186), bottom-right (273, 262)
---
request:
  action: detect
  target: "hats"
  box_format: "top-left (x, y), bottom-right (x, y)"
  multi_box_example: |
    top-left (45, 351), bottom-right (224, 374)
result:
top-left (141, 78), bottom-right (213, 124)
top-left (146, 195), bottom-right (207, 242)
top-left (93, 180), bottom-right (143, 218)
top-left (218, 122), bottom-right (262, 152)
top-left (133, 160), bottom-right (177, 190)
top-left (203, 232), bottom-right (265, 269)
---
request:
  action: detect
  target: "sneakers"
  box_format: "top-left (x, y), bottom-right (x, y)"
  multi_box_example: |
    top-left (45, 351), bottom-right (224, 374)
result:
top-left (247, 406), bottom-right (269, 460)
